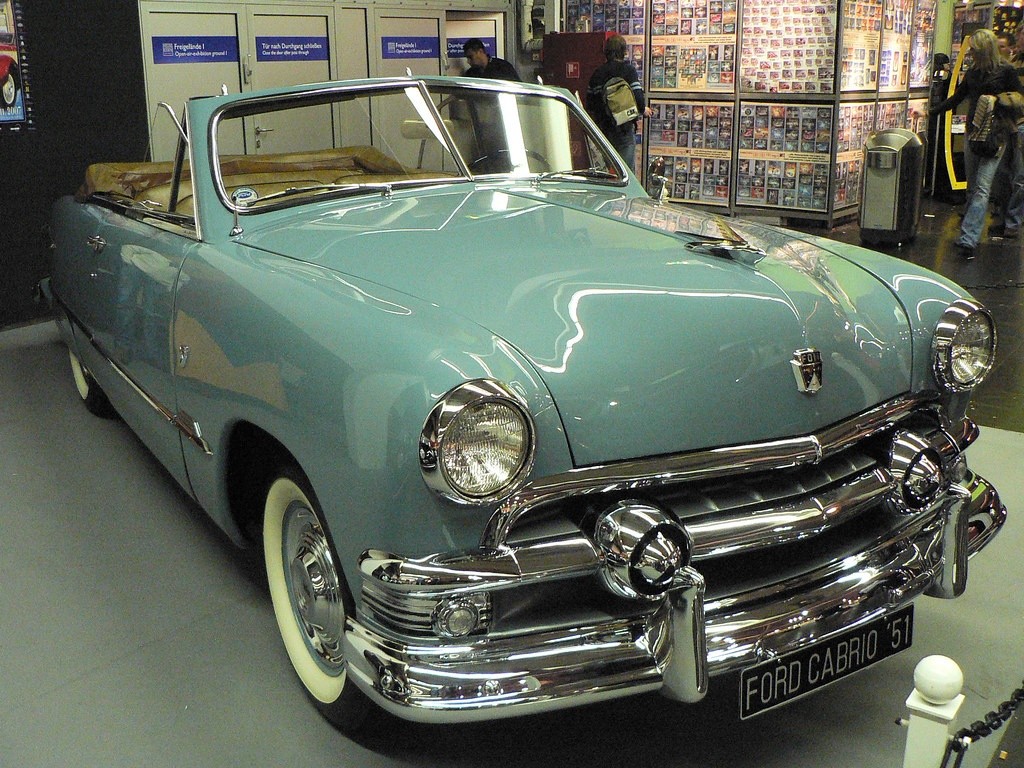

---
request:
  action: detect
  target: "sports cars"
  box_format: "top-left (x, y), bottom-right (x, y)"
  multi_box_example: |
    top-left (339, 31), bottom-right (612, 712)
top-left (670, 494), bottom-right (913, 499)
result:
top-left (38, 70), bottom-right (1011, 733)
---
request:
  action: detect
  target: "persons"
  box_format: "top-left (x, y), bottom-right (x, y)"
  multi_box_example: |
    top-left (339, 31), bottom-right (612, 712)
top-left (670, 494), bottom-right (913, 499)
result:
top-left (585, 33), bottom-right (653, 176)
top-left (434, 38), bottom-right (522, 174)
top-left (912, 18), bottom-right (1024, 259)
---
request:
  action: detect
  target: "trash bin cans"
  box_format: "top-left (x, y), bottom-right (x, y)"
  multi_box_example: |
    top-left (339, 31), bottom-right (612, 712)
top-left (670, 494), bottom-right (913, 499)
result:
top-left (854, 127), bottom-right (927, 247)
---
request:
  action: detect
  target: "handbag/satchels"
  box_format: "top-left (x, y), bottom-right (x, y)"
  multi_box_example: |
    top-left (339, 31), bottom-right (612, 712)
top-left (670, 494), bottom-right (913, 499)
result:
top-left (601, 75), bottom-right (640, 128)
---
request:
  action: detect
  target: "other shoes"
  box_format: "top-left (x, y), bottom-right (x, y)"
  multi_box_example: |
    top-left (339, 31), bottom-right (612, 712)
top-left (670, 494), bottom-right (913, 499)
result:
top-left (1004, 226), bottom-right (1019, 238)
top-left (987, 220), bottom-right (1008, 235)
top-left (955, 239), bottom-right (979, 255)
